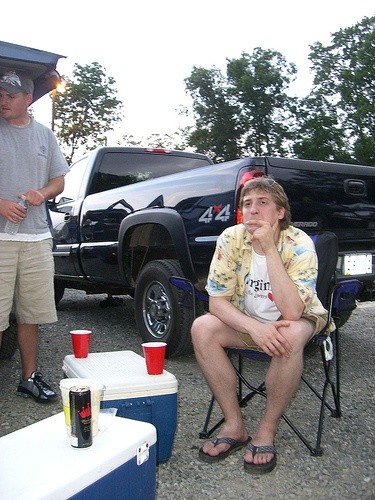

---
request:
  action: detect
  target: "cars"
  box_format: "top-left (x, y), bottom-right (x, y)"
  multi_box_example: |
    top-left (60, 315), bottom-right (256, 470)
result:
top-left (0, 39), bottom-right (68, 362)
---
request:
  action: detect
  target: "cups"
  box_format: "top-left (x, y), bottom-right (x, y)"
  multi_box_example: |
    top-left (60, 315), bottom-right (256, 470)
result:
top-left (70, 330), bottom-right (91, 358)
top-left (58, 378), bottom-right (105, 432)
top-left (141, 342), bottom-right (167, 376)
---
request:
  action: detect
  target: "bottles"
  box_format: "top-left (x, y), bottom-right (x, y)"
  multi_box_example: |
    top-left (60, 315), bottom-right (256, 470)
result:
top-left (3, 195), bottom-right (26, 236)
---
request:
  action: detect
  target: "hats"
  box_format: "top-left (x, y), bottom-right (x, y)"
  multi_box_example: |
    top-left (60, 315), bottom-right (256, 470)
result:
top-left (0, 70), bottom-right (34, 96)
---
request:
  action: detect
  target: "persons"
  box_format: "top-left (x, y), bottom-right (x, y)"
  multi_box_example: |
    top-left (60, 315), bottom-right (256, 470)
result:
top-left (191, 177), bottom-right (336, 474)
top-left (0, 68), bottom-right (71, 403)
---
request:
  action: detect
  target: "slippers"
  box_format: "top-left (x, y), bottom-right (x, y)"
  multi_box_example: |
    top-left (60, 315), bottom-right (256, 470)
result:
top-left (245, 440), bottom-right (277, 474)
top-left (198, 435), bottom-right (253, 463)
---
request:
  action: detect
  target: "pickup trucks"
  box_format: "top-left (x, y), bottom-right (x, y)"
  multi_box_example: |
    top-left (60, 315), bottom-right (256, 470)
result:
top-left (47, 146), bottom-right (375, 360)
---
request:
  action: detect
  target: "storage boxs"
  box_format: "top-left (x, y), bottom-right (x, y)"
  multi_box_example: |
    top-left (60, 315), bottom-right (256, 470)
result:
top-left (62, 351), bottom-right (177, 466)
top-left (0, 411), bottom-right (157, 500)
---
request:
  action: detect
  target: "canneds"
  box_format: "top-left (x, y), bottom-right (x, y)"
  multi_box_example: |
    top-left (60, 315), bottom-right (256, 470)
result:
top-left (68, 386), bottom-right (92, 449)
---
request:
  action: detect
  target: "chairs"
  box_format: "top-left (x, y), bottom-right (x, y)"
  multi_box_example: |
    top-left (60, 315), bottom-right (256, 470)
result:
top-left (169, 232), bottom-right (362, 457)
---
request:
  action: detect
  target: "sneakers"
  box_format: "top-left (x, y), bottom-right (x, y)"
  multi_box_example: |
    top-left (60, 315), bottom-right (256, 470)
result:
top-left (17, 371), bottom-right (58, 402)
